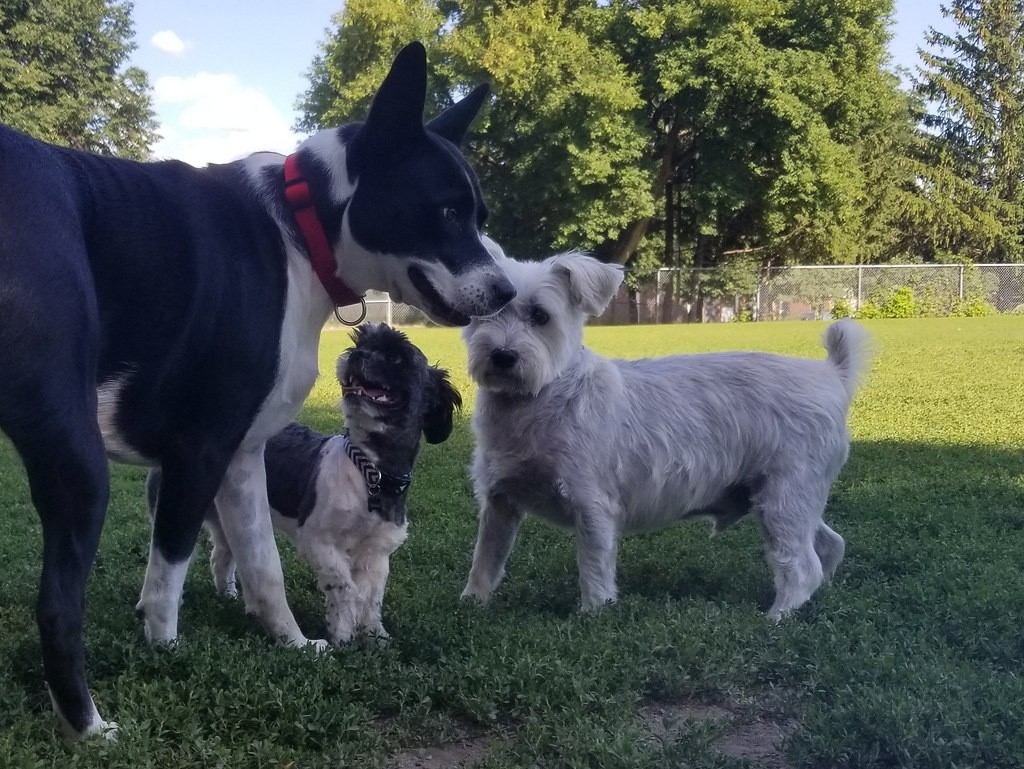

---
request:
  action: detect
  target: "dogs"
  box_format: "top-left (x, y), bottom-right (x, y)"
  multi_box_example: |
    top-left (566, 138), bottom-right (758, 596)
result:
top-left (0, 40), bottom-right (517, 747)
top-left (458, 234), bottom-right (877, 624)
